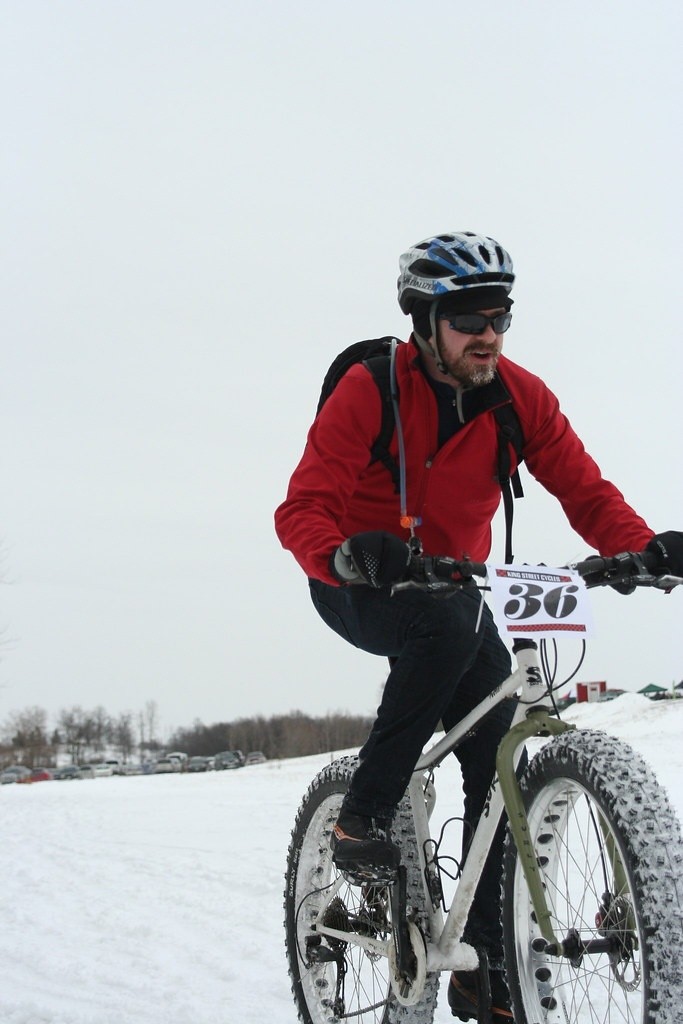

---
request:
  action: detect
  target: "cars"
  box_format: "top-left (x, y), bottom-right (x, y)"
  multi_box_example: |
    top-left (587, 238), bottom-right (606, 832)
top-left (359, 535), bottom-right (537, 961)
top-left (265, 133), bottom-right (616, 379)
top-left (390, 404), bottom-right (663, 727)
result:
top-left (245, 752), bottom-right (266, 766)
top-left (0, 752), bottom-right (244, 785)
top-left (215, 751), bottom-right (240, 770)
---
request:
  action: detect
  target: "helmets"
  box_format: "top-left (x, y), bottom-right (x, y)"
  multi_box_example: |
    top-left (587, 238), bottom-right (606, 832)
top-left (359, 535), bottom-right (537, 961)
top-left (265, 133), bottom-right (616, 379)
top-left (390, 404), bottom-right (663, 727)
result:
top-left (397, 233), bottom-right (515, 316)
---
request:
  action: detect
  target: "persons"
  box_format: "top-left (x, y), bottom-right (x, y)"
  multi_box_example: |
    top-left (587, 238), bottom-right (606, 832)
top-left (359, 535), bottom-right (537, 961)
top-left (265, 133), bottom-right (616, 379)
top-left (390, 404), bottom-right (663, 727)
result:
top-left (273, 230), bottom-right (682, 1023)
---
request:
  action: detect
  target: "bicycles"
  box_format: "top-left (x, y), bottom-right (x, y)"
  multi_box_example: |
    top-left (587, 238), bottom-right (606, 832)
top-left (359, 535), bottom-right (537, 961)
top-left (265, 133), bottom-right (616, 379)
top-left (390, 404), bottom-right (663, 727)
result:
top-left (282, 540), bottom-right (683, 1024)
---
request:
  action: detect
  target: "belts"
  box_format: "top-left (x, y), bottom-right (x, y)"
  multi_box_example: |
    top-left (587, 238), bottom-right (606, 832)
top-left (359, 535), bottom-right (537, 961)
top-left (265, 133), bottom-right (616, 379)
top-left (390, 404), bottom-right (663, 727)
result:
top-left (414, 556), bottom-right (488, 583)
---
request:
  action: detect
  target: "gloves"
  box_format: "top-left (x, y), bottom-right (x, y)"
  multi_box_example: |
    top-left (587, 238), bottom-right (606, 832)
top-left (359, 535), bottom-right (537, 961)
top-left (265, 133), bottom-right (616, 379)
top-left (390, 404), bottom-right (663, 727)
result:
top-left (647, 530), bottom-right (683, 577)
top-left (327, 530), bottom-right (413, 590)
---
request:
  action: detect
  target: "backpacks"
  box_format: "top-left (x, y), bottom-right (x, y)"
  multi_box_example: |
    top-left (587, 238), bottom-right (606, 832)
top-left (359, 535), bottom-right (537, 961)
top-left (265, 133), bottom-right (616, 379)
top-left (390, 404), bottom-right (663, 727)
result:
top-left (314, 335), bottom-right (526, 467)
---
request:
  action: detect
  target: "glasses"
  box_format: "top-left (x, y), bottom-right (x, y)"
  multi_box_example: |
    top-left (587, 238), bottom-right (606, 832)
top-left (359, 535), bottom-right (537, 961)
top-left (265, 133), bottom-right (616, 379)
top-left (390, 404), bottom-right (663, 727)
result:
top-left (437, 311), bottom-right (512, 335)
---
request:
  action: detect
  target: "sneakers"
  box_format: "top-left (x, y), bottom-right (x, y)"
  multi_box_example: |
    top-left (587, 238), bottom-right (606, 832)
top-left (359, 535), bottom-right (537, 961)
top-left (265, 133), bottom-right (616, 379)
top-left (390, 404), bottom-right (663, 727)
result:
top-left (447, 968), bottom-right (515, 1024)
top-left (329, 820), bottom-right (402, 864)
